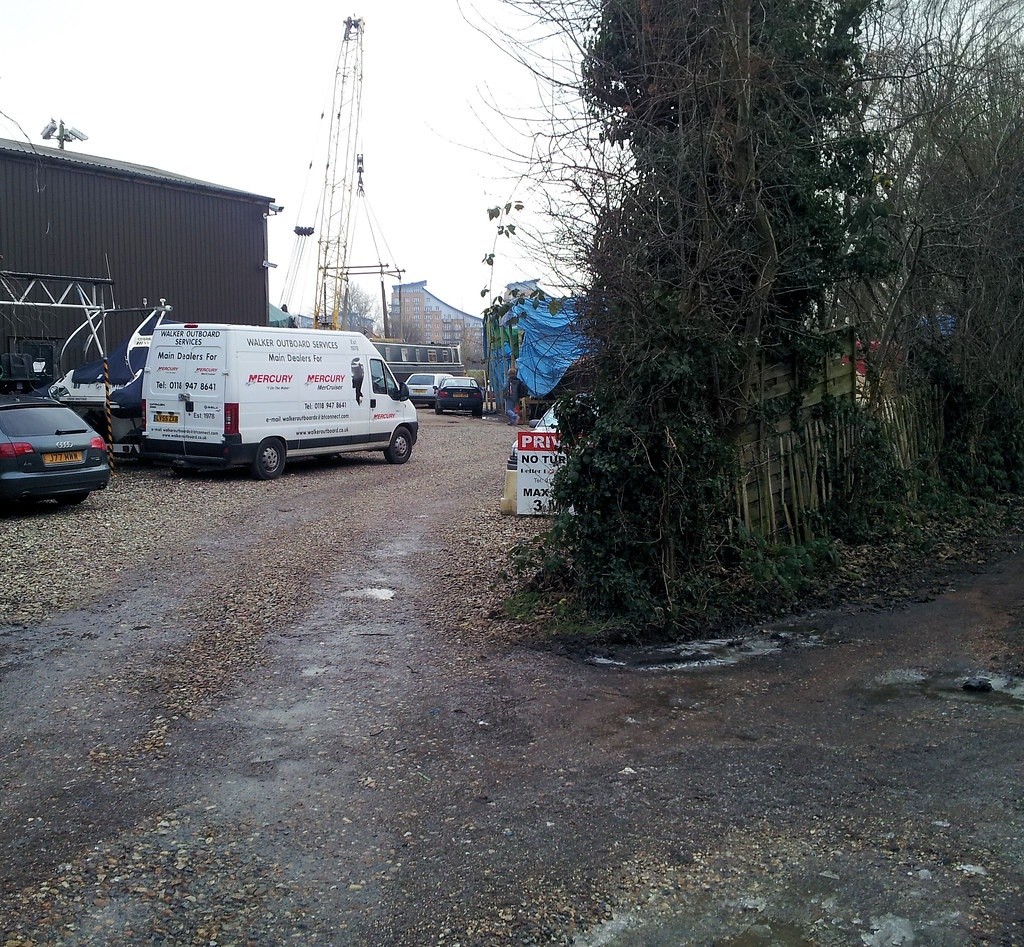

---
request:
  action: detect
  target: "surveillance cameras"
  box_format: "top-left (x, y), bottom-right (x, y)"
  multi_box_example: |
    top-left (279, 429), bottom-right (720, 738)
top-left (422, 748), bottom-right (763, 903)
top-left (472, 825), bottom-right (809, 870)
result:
top-left (263, 261), bottom-right (278, 268)
top-left (64, 129), bottom-right (73, 142)
top-left (41, 122), bottom-right (57, 139)
top-left (269, 202), bottom-right (284, 212)
top-left (69, 128), bottom-right (88, 141)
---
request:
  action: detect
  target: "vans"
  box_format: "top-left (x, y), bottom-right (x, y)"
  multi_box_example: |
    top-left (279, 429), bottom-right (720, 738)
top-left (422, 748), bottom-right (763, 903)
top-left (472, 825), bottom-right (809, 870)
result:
top-left (141, 323), bottom-right (419, 481)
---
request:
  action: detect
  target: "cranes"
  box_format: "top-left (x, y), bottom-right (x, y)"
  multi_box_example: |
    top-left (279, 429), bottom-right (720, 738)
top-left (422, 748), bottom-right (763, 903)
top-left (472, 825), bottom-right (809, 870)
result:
top-left (314, 11), bottom-right (406, 341)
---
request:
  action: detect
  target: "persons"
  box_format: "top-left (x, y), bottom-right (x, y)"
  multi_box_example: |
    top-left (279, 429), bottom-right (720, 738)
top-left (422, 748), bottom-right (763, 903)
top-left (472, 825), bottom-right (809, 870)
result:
top-left (503, 368), bottom-right (521, 426)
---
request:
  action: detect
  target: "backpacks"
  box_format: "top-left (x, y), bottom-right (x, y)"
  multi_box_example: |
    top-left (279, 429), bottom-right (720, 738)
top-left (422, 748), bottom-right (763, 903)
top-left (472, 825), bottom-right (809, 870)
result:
top-left (517, 378), bottom-right (528, 398)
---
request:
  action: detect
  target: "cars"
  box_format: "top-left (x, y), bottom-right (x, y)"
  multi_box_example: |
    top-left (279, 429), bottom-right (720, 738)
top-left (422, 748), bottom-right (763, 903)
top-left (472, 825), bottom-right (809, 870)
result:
top-left (432, 376), bottom-right (485, 417)
top-left (403, 373), bottom-right (455, 409)
top-left (0, 395), bottom-right (111, 506)
top-left (507, 394), bottom-right (603, 470)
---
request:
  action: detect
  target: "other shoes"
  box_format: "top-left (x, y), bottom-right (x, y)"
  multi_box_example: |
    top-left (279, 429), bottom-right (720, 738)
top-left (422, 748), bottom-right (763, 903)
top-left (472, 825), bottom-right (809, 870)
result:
top-left (514, 415), bottom-right (520, 426)
top-left (503, 394), bottom-right (506, 399)
top-left (508, 422), bottom-right (510, 425)
